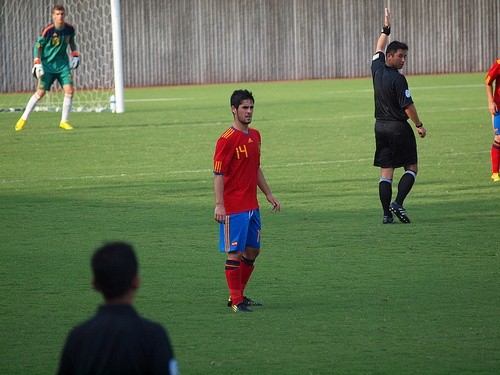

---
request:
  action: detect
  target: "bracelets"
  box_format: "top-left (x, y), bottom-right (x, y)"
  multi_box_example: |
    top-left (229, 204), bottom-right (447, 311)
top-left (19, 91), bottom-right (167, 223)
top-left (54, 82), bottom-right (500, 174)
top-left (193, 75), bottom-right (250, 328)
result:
top-left (381, 25), bottom-right (391, 36)
top-left (416, 123), bottom-right (422, 128)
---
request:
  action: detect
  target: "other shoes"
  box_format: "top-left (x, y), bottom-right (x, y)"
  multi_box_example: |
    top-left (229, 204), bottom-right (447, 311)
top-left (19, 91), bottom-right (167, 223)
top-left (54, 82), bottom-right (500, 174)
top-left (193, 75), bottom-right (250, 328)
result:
top-left (491, 172), bottom-right (500, 182)
top-left (59, 120), bottom-right (72, 130)
top-left (232, 302), bottom-right (253, 313)
top-left (227, 295), bottom-right (262, 306)
top-left (15, 117), bottom-right (26, 131)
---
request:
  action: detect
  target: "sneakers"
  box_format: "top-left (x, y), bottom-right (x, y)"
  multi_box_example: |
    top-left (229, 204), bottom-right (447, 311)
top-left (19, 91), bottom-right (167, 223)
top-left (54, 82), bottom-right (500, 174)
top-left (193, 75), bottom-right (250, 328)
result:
top-left (383, 215), bottom-right (394, 224)
top-left (389, 200), bottom-right (410, 223)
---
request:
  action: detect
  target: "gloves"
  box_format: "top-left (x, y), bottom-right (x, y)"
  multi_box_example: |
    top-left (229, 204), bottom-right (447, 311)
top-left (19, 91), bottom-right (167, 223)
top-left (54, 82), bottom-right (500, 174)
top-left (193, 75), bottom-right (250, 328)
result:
top-left (69, 52), bottom-right (79, 69)
top-left (31, 60), bottom-right (44, 79)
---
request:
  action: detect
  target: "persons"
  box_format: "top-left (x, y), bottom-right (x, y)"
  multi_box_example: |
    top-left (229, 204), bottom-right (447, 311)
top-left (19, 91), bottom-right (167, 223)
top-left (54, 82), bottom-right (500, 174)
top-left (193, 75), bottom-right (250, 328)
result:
top-left (14, 5), bottom-right (80, 130)
top-left (58, 242), bottom-right (180, 375)
top-left (485, 59), bottom-right (500, 182)
top-left (213, 89), bottom-right (280, 312)
top-left (371, 8), bottom-right (427, 223)
